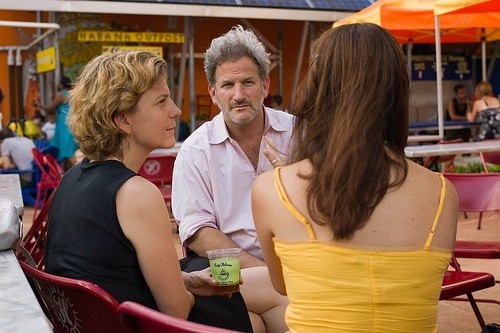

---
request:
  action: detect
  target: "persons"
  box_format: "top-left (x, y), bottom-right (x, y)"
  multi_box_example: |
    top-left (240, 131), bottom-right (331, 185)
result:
top-left (41, 48), bottom-right (291, 331)
top-left (444, 84), bottom-right (473, 140)
top-left (268, 94), bottom-right (288, 112)
top-left (1, 72), bottom-right (80, 192)
top-left (247, 22), bottom-right (462, 333)
top-left (463, 82), bottom-right (500, 140)
top-left (170, 23), bottom-right (311, 271)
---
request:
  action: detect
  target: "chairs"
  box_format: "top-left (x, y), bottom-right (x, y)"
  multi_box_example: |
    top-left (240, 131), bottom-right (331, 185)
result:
top-left (440, 172), bottom-right (500, 307)
top-left (0, 168), bottom-right (39, 207)
top-left (16, 205), bottom-right (48, 269)
top-left (30, 148), bottom-right (60, 222)
top-left (18, 118), bottom-right (31, 139)
top-left (408, 120), bottom-right (483, 132)
top-left (136, 153), bottom-right (179, 233)
top-left (42, 154), bottom-right (64, 210)
top-left (423, 154), bottom-right (469, 219)
top-left (18, 259), bottom-right (120, 333)
top-left (0, 120), bottom-right (18, 141)
top-left (117, 300), bottom-right (242, 333)
top-left (435, 249), bottom-right (496, 333)
top-left (30, 147), bottom-right (59, 198)
top-left (477, 150), bottom-right (500, 229)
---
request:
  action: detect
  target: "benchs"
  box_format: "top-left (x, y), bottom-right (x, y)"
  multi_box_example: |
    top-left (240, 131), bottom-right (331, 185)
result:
top-left (406, 139), bottom-right (500, 158)
top-left (407, 135), bottom-right (443, 142)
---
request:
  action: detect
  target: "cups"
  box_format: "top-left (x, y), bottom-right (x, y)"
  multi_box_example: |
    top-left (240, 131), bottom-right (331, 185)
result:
top-left (206, 248), bottom-right (242, 294)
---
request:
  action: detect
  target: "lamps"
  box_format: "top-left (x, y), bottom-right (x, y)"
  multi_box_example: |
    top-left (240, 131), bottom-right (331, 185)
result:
top-left (15, 49), bottom-right (23, 66)
top-left (7, 49), bottom-right (15, 66)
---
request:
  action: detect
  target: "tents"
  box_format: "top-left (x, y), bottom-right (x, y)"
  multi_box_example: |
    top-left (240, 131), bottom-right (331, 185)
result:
top-left (332, 0), bottom-right (500, 144)
